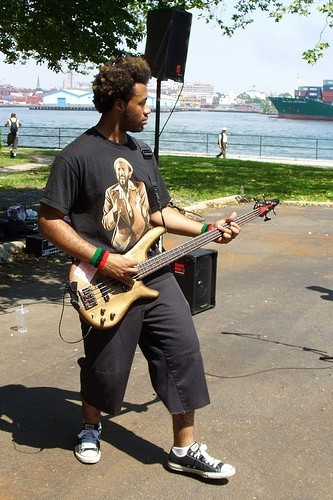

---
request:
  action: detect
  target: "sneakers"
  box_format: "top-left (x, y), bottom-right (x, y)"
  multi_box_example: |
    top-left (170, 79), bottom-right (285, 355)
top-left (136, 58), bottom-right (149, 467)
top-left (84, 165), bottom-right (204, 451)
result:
top-left (167, 442), bottom-right (235, 479)
top-left (74, 420), bottom-right (102, 464)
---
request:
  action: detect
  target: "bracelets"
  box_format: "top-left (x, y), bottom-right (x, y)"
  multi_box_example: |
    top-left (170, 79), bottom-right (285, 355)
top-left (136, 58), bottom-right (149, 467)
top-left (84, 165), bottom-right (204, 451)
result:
top-left (201, 223), bottom-right (213, 233)
top-left (88, 247), bottom-right (111, 269)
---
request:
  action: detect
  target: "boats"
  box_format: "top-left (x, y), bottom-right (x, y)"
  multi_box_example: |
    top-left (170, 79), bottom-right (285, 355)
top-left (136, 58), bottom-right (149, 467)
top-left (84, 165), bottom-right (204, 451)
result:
top-left (267, 79), bottom-right (333, 121)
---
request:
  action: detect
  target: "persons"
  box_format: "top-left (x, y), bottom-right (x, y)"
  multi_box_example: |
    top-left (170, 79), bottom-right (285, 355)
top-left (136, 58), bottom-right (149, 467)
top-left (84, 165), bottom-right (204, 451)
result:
top-left (216, 127), bottom-right (227, 158)
top-left (102, 158), bottom-right (151, 249)
top-left (36, 54), bottom-right (241, 479)
top-left (4, 112), bottom-right (21, 157)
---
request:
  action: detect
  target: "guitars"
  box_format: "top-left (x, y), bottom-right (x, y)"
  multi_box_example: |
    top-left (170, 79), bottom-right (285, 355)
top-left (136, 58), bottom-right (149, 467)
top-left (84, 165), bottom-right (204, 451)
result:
top-left (68, 197), bottom-right (281, 329)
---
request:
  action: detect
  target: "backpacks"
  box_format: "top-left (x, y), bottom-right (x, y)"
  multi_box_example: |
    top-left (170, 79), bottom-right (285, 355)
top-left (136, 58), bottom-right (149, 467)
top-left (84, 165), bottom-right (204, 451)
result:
top-left (9, 118), bottom-right (18, 133)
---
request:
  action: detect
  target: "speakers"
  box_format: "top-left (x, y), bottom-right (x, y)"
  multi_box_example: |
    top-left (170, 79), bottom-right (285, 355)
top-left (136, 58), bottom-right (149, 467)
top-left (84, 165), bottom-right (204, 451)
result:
top-left (143, 7), bottom-right (193, 84)
top-left (170, 248), bottom-right (218, 316)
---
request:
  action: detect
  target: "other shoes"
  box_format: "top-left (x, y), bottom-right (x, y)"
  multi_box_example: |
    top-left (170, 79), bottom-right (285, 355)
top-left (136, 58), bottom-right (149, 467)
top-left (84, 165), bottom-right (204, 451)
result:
top-left (11, 153), bottom-right (15, 157)
